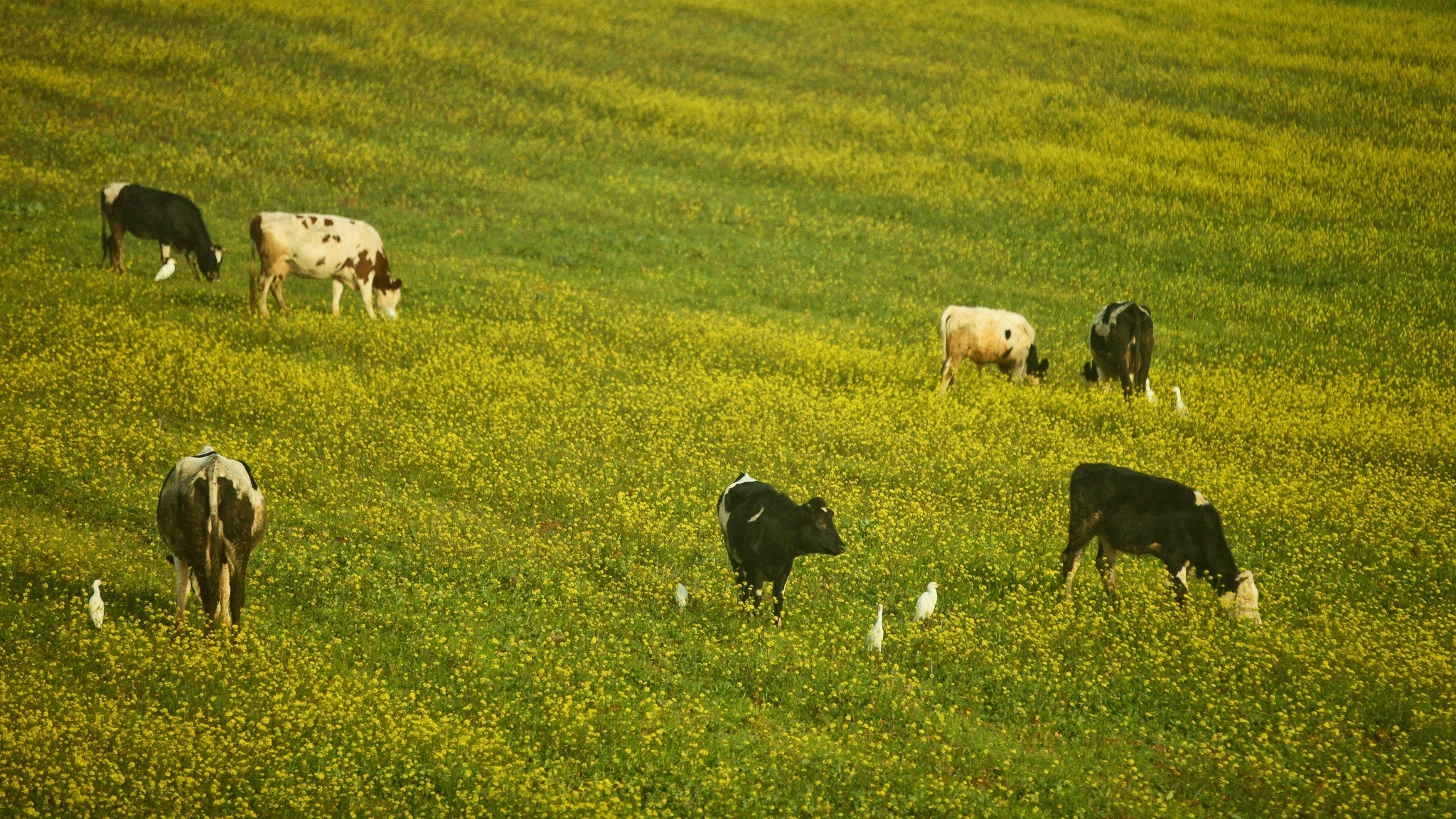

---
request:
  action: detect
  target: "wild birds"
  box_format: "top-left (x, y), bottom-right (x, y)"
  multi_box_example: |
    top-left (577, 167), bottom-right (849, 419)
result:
top-left (154, 258), bottom-right (177, 281)
top-left (1171, 386), bottom-right (1189, 418)
top-left (1145, 378), bottom-right (1156, 406)
top-left (88, 579), bottom-right (104, 628)
top-left (676, 583), bottom-right (688, 607)
top-left (914, 582), bottom-right (942, 629)
top-left (866, 603), bottom-right (884, 657)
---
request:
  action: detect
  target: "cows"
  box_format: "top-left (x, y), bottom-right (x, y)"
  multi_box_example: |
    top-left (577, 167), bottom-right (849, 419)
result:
top-left (157, 446), bottom-right (267, 648)
top-left (932, 305), bottom-right (1049, 398)
top-left (717, 472), bottom-right (847, 631)
top-left (99, 182), bottom-right (223, 283)
top-left (1080, 302), bottom-right (1154, 418)
top-left (1061, 463), bottom-right (1262, 628)
top-left (250, 212), bottom-right (402, 320)
